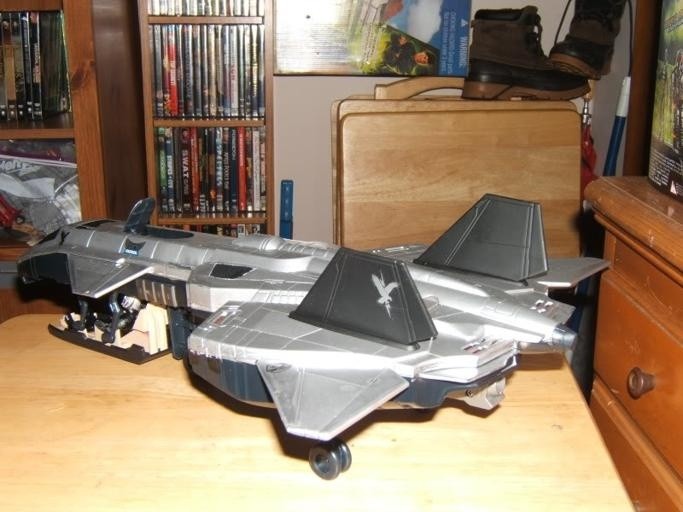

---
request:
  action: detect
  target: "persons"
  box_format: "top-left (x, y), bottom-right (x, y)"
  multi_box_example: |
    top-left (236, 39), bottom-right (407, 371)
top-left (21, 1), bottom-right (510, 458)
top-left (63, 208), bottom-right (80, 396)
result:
top-left (385, 34), bottom-right (435, 76)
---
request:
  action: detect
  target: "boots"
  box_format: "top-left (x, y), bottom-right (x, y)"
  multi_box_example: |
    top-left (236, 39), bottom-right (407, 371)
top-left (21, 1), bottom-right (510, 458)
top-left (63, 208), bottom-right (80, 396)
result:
top-left (551, 1), bottom-right (625, 81)
top-left (462, 5), bottom-right (592, 102)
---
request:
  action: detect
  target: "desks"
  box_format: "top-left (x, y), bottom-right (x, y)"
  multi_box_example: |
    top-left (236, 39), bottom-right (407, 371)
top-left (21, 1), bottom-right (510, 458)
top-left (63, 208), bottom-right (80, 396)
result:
top-left (0, 313), bottom-right (636, 511)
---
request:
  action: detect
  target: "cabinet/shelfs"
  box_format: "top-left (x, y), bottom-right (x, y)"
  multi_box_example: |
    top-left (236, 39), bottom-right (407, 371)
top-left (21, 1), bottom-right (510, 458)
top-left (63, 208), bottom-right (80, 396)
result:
top-left (138, 0), bottom-right (275, 239)
top-left (585, 175), bottom-right (683, 511)
top-left (0, 0), bottom-right (145, 327)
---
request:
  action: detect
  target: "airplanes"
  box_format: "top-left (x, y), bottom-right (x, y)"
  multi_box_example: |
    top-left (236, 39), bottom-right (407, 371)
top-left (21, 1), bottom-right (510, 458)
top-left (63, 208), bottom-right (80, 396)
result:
top-left (14, 191), bottom-right (611, 481)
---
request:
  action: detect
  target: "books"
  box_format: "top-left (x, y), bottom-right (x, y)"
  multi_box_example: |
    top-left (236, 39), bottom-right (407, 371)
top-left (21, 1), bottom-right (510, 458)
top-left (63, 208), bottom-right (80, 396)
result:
top-left (145, 0), bottom-right (266, 237)
top-left (0, 10), bottom-right (73, 121)
